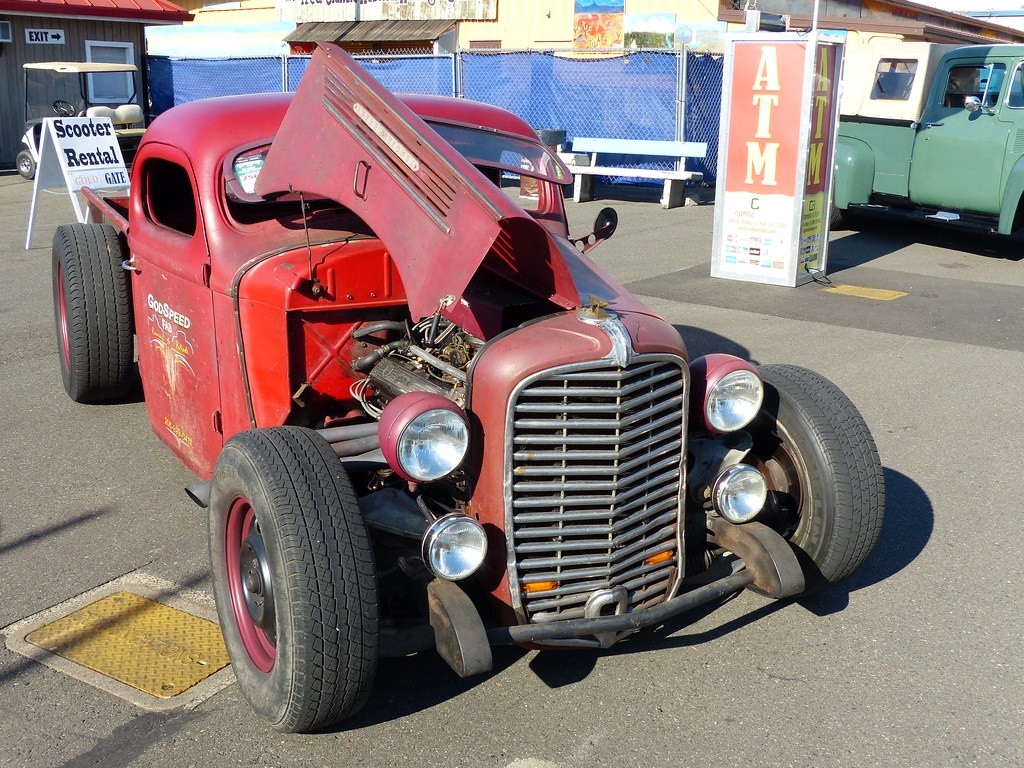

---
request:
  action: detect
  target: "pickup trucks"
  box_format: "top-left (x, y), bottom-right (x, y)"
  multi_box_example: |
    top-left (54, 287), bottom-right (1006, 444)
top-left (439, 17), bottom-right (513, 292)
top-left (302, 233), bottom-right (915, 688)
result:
top-left (824, 37), bottom-right (1023, 246)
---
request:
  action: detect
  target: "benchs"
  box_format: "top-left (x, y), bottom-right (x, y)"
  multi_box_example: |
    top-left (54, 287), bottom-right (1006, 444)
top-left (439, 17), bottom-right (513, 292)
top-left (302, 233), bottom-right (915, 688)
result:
top-left (562, 137), bottom-right (708, 209)
top-left (85, 105), bottom-right (147, 138)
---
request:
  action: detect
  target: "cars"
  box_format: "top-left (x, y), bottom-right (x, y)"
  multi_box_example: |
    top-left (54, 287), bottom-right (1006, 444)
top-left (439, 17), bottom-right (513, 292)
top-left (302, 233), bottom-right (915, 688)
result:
top-left (53, 40), bottom-right (887, 733)
